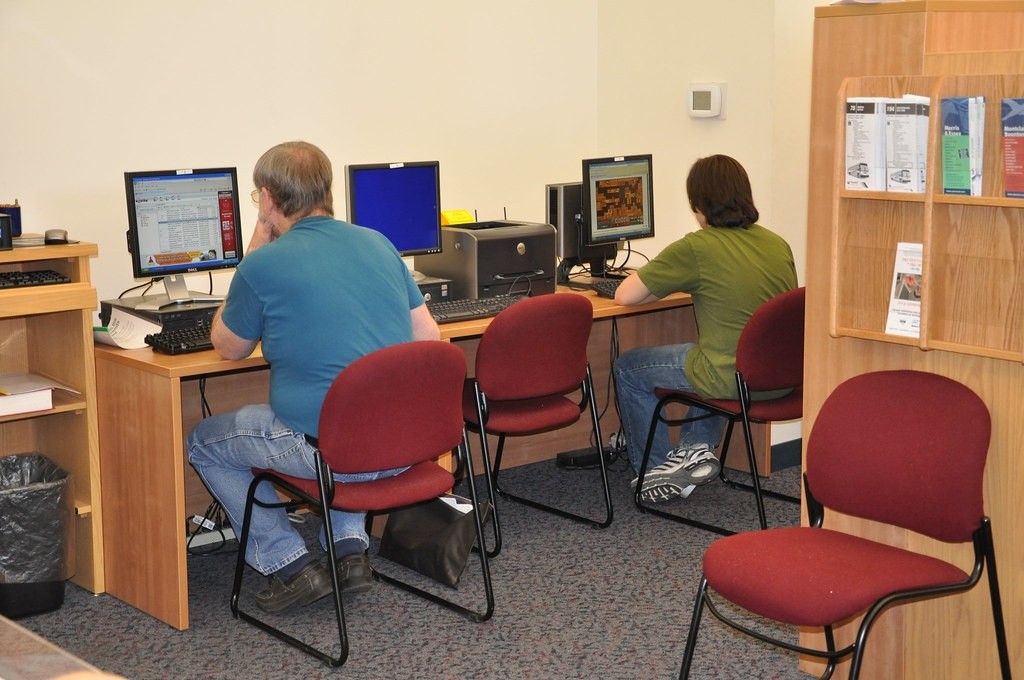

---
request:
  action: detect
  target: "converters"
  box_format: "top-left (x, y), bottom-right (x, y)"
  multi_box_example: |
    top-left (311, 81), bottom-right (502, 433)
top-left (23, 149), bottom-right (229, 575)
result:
top-left (557, 446), bottom-right (613, 468)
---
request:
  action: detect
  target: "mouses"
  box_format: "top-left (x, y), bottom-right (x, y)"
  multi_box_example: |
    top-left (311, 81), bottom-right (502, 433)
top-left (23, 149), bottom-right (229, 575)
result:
top-left (44, 229), bottom-right (69, 244)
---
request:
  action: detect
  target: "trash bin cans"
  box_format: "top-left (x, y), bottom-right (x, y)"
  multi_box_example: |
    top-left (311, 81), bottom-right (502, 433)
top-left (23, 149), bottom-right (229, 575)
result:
top-left (0, 453), bottom-right (72, 619)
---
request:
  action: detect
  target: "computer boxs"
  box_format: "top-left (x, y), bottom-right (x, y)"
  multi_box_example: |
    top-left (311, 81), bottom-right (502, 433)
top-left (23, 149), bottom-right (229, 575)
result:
top-left (99, 290), bottom-right (225, 333)
top-left (414, 277), bottom-right (453, 305)
top-left (546, 183), bottom-right (617, 261)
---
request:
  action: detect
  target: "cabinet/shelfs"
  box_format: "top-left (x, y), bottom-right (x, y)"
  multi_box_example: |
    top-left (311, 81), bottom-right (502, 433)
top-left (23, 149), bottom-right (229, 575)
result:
top-left (800, 1), bottom-right (1024, 679)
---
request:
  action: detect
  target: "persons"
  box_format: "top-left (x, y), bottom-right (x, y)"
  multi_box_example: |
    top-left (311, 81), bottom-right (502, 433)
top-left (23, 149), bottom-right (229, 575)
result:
top-left (612, 155), bottom-right (800, 501)
top-left (185, 141), bottom-right (442, 613)
top-left (202, 250), bottom-right (217, 261)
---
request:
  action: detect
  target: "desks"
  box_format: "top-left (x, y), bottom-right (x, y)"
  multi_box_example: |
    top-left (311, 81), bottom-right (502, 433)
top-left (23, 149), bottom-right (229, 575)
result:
top-left (0, 241), bottom-right (105, 602)
top-left (93, 264), bottom-right (774, 632)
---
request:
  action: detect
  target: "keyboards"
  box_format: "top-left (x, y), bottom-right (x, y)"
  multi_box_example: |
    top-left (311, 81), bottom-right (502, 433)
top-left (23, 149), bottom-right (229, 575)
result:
top-left (144, 326), bottom-right (214, 355)
top-left (427, 293), bottom-right (520, 324)
top-left (590, 279), bottom-right (623, 299)
top-left (0, 270), bottom-right (71, 289)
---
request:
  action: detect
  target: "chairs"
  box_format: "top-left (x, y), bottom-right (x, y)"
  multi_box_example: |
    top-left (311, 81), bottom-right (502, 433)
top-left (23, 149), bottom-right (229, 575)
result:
top-left (428, 294), bottom-right (614, 556)
top-left (634, 286), bottom-right (809, 530)
top-left (681, 371), bottom-right (1013, 680)
top-left (228, 341), bottom-right (495, 668)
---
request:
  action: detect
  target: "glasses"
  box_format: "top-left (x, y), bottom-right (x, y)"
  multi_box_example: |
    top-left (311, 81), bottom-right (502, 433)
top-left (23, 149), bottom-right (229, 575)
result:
top-left (251, 187), bottom-right (271, 203)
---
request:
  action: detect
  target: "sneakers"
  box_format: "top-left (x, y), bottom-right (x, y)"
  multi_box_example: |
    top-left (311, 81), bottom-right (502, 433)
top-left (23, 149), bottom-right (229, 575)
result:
top-left (629, 445), bottom-right (721, 503)
top-left (326, 553), bottom-right (372, 594)
top-left (256, 560), bottom-right (333, 614)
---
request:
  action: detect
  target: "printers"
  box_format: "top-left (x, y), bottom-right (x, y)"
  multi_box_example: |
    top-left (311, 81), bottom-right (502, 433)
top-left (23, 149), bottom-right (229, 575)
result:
top-left (414, 219), bottom-right (557, 297)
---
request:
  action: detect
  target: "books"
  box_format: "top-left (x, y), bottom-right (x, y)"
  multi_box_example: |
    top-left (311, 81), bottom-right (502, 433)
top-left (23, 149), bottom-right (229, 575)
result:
top-left (0, 387), bottom-right (55, 416)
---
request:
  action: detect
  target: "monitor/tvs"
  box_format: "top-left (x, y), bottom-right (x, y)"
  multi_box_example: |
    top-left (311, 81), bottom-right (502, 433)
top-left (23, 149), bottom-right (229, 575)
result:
top-left (344, 161), bottom-right (443, 281)
top-left (124, 166), bottom-right (243, 310)
top-left (581, 153), bottom-right (655, 246)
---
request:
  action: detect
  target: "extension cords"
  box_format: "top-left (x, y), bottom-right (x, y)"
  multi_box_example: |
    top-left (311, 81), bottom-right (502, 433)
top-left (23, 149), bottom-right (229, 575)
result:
top-left (186, 527), bottom-right (236, 548)
